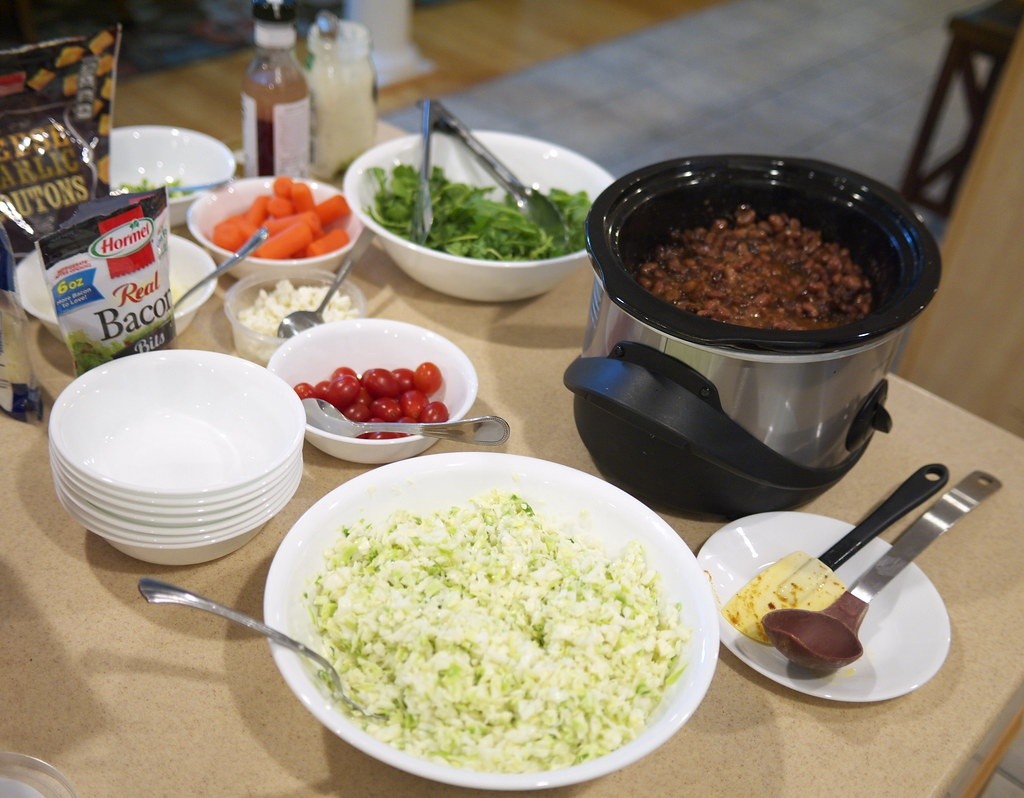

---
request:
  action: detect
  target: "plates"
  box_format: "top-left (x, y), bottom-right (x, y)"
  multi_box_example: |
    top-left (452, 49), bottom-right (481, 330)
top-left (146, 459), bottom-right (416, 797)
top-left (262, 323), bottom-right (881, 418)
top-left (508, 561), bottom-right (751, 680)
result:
top-left (694, 512), bottom-right (952, 705)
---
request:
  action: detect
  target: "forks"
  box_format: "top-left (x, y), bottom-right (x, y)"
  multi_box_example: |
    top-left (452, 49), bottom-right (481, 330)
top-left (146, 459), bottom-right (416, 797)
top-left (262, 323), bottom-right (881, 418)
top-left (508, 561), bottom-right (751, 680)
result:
top-left (134, 579), bottom-right (373, 717)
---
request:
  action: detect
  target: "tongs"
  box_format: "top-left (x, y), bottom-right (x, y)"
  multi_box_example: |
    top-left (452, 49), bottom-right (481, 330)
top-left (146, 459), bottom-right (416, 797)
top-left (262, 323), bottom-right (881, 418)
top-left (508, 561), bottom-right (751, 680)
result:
top-left (411, 95), bottom-right (569, 248)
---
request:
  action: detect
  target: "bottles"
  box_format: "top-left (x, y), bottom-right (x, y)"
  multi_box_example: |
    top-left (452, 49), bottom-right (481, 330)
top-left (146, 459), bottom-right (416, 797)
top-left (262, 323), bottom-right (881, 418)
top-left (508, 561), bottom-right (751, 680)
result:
top-left (240, 0), bottom-right (315, 176)
top-left (302, 14), bottom-right (379, 183)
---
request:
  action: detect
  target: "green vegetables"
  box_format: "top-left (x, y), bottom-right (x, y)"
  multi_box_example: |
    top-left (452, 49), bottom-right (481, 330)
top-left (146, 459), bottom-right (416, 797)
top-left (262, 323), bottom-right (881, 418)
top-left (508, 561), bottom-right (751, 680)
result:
top-left (362, 163), bottom-right (593, 262)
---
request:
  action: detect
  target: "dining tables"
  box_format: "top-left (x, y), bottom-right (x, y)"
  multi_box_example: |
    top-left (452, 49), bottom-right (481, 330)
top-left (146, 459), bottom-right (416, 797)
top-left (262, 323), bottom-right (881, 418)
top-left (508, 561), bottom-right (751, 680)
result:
top-left (2, 121), bottom-right (1024, 798)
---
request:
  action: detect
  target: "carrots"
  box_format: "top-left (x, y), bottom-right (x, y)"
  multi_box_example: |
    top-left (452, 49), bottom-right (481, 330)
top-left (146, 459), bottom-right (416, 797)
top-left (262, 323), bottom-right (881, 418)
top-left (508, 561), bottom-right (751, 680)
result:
top-left (214, 177), bottom-right (352, 259)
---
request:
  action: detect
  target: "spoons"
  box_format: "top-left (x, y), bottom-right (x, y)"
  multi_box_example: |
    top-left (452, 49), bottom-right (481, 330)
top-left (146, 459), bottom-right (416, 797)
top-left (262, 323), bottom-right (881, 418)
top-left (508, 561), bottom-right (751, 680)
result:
top-left (299, 395), bottom-right (510, 446)
top-left (277, 259), bottom-right (357, 339)
top-left (759, 466), bottom-right (1002, 667)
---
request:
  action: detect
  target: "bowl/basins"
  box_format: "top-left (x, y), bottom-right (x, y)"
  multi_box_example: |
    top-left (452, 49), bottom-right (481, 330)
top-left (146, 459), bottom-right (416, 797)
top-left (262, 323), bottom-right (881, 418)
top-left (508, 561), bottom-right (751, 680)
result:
top-left (108, 124), bottom-right (237, 229)
top-left (268, 318), bottom-right (480, 465)
top-left (186, 176), bottom-right (363, 287)
top-left (259, 448), bottom-right (722, 790)
top-left (341, 129), bottom-right (616, 300)
top-left (222, 266), bottom-right (367, 370)
top-left (47, 350), bottom-right (306, 568)
top-left (11, 234), bottom-right (217, 344)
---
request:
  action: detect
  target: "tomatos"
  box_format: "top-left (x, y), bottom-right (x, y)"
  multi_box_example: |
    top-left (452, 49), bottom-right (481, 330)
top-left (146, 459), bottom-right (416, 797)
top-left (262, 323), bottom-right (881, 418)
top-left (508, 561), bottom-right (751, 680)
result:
top-left (293, 360), bottom-right (450, 441)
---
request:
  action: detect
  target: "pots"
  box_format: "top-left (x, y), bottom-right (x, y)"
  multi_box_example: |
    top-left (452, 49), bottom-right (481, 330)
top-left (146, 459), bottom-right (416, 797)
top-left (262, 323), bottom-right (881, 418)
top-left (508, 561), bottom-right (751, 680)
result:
top-left (563, 146), bottom-right (944, 528)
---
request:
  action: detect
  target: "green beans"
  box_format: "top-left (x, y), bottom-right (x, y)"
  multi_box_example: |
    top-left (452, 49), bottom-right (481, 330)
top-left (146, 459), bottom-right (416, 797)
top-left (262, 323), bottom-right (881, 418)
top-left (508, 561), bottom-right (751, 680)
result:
top-left (633, 208), bottom-right (874, 331)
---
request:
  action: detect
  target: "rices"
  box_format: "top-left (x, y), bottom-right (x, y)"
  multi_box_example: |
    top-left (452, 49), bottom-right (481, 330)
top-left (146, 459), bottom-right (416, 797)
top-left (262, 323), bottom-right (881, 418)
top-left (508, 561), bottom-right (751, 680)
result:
top-left (300, 485), bottom-right (692, 776)
